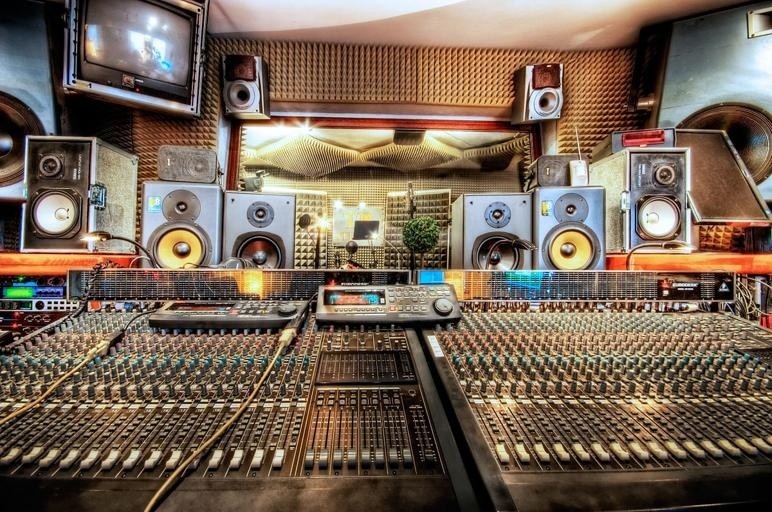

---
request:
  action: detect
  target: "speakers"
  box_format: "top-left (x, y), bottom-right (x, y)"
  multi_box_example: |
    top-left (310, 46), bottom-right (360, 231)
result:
top-left (511, 63), bottom-right (564, 125)
top-left (221, 54), bottom-right (271, 120)
top-left (142, 145), bottom-right (297, 269)
top-left (451, 147), bottom-right (691, 272)
top-left (644, 0), bottom-right (772, 204)
top-left (19, 136), bottom-right (140, 254)
top-left (0, 0), bottom-right (62, 207)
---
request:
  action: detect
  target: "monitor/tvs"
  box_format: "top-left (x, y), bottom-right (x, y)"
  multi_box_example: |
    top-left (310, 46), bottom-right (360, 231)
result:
top-left (77, 0), bottom-right (196, 105)
top-left (676, 129), bottom-right (772, 227)
